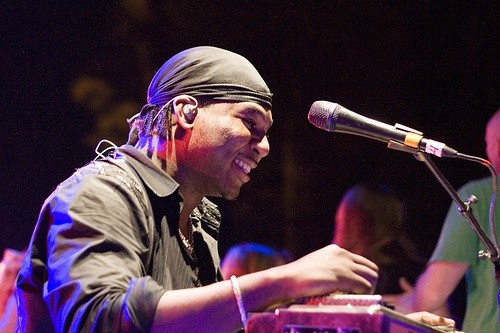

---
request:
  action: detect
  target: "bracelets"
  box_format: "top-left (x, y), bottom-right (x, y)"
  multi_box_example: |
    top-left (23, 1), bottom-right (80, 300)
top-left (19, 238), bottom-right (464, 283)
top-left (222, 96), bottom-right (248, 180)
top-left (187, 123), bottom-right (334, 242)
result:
top-left (229, 276), bottom-right (248, 324)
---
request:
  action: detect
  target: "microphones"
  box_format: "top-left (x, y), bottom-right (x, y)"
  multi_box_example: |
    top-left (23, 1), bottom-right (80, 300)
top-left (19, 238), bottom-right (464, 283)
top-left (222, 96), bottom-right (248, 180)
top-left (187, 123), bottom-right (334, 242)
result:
top-left (308, 101), bottom-right (457, 157)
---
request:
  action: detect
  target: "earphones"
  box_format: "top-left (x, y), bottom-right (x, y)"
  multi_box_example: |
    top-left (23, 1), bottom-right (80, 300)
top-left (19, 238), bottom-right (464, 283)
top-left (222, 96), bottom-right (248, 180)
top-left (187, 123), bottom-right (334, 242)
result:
top-left (183, 103), bottom-right (198, 121)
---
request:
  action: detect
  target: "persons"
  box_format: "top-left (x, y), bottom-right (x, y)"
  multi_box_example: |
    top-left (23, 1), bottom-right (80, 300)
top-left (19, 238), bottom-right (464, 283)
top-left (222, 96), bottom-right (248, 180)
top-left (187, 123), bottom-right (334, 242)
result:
top-left (0, 45), bottom-right (380, 333)
top-left (222, 108), bottom-right (500, 333)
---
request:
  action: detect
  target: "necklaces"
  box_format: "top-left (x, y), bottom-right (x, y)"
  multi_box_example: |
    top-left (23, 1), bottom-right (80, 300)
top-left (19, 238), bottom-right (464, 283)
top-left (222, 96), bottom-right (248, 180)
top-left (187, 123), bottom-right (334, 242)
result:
top-left (178, 217), bottom-right (195, 256)
